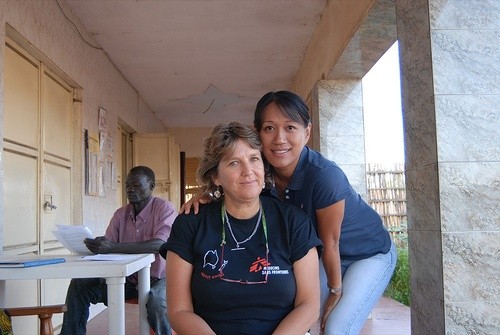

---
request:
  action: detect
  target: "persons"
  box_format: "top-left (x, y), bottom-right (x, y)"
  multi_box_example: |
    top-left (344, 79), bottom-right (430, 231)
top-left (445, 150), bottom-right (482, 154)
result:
top-left (177, 91), bottom-right (398, 335)
top-left (158, 120), bottom-right (325, 335)
top-left (57, 166), bottom-right (179, 335)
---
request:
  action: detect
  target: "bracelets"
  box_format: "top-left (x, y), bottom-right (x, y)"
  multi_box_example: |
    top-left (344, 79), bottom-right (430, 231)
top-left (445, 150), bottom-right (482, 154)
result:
top-left (326, 283), bottom-right (342, 297)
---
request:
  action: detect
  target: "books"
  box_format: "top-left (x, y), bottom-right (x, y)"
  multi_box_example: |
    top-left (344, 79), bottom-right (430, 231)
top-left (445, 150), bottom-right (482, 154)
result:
top-left (0, 257), bottom-right (66, 270)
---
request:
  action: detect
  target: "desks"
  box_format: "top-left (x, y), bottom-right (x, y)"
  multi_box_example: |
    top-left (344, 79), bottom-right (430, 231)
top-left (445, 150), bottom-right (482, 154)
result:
top-left (0, 252), bottom-right (155, 334)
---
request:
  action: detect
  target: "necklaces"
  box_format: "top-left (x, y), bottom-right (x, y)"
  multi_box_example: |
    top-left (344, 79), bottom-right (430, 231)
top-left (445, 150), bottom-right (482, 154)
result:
top-left (224, 202), bottom-right (262, 252)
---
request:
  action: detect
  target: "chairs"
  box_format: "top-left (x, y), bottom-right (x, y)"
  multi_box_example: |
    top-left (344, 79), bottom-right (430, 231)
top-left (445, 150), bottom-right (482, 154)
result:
top-left (2, 305), bottom-right (67, 335)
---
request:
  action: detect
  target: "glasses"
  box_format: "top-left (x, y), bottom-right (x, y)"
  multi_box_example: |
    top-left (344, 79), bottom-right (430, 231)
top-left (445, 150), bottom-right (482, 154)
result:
top-left (220, 242), bottom-right (269, 285)
